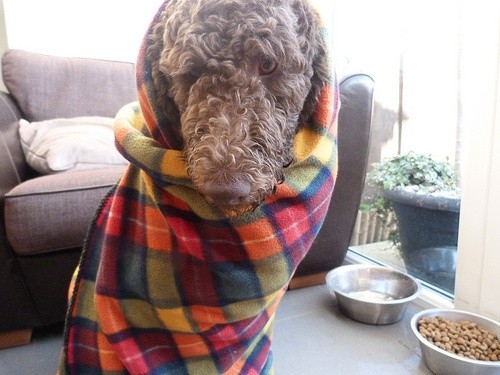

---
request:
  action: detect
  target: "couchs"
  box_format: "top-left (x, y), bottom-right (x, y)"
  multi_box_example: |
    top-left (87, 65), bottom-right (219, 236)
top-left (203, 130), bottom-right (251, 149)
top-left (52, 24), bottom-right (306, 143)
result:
top-left (1, 50), bottom-right (374, 348)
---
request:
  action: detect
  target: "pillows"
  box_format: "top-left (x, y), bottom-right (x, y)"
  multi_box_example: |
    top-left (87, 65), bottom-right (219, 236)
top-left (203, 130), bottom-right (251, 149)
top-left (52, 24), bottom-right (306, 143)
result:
top-left (16, 115), bottom-right (132, 173)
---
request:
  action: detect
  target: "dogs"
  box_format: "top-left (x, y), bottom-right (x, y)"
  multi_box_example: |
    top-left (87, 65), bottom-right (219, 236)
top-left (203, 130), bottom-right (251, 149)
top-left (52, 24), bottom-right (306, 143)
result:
top-left (59, 0), bottom-right (344, 374)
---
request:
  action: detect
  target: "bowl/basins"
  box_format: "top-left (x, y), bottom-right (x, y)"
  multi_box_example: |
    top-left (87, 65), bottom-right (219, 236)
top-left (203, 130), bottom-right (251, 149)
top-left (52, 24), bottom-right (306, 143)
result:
top-left (412, 310), bottom-right (500, 374)
top-left (325, 263), bottom-right (420, 326)
